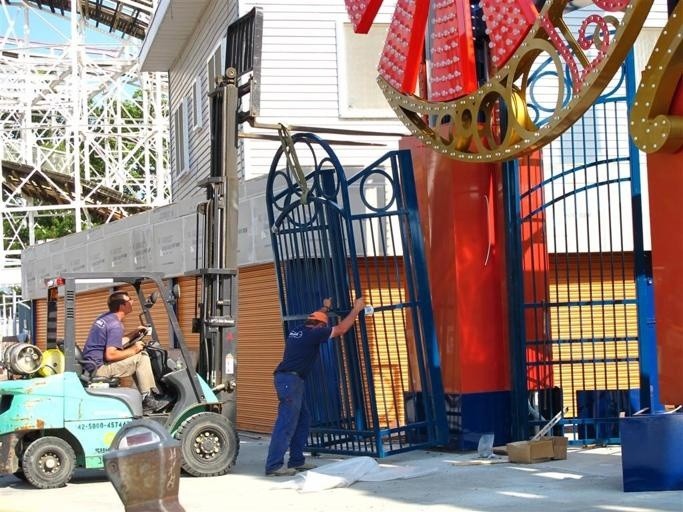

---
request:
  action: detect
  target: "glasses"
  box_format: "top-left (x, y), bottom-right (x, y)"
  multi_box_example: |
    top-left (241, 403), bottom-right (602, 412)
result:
top-left (124, 296), bottom-right (133, 303)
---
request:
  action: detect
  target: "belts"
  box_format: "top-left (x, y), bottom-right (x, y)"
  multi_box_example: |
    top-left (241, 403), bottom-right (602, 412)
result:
top-left (286, 370), bottom-right (308, 379)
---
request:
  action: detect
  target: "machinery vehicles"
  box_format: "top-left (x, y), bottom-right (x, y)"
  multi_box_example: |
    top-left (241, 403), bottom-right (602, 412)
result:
top-left (1, 7), bottom-right (409, 489)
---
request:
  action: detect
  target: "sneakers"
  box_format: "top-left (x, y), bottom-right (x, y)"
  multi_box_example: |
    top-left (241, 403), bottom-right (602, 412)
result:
top-left (266, 464), bottom-right (297, 476)
top-left (143, 397), bottom-right (169, 415)
top-left (295, 464), bottom-right (318, 472)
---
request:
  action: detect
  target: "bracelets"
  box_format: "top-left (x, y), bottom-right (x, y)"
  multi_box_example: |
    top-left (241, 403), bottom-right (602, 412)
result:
top-left (323, 306), bottom-right (329, 312)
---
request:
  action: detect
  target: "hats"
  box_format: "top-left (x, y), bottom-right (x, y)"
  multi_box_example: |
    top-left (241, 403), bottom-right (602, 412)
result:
top-left (307, 311), bottom-right (330, 327)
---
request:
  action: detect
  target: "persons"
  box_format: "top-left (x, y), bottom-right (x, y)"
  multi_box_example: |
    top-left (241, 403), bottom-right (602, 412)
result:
top-left (81, 291), bottom-right (175, 415)
top-left (264, 297), bottom-right (366, 477)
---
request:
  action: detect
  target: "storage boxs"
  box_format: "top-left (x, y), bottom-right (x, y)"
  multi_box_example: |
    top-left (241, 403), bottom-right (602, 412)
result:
top-left (505, 440), bottom-right (554, 465)
top-left (539, 436), bottom-right (569, 461)
